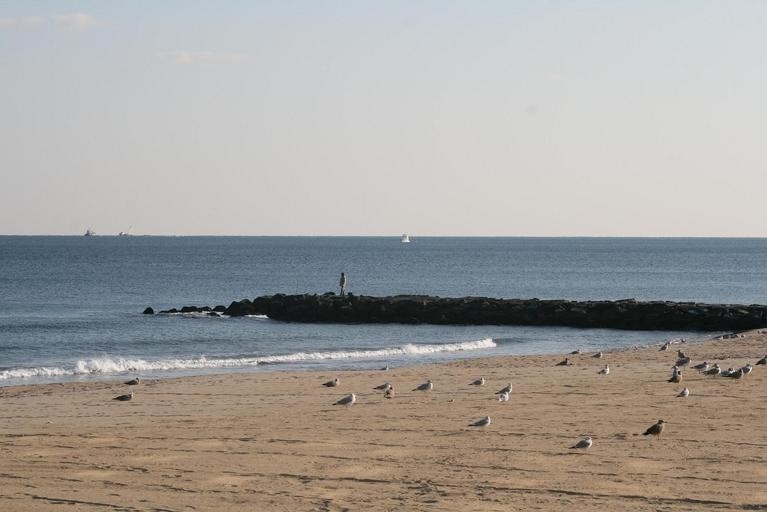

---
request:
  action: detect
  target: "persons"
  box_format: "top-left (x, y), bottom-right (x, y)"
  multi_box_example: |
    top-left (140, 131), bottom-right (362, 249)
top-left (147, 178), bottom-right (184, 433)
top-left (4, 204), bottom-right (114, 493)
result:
top-left (338, 272), bottom-right (346, 296)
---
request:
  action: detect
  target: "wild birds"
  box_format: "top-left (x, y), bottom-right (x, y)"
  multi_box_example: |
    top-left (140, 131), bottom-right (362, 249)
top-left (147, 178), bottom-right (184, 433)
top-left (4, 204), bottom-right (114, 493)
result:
top-left (642, 420), bottom-right (665, 440)
top-left (112, 377), bottom-right (140, 401)
top-left (496, 382), bottom-right (513, 404)
top-left (322, 378), bottom-right (433, 405)
top-left (657, 330), bottom-right (767, 398)
top-left (380, 365), bottom-right (388, 370)
top-left (567, 436), bottom-right (592, 448)
top-left (467, 376), bottom-right (485, 385)
top-left (554, 349), bottom-right (608, 375)
top-left (467, 415), bottom-right (490, 426)
